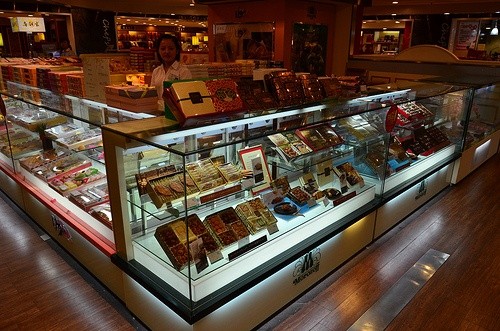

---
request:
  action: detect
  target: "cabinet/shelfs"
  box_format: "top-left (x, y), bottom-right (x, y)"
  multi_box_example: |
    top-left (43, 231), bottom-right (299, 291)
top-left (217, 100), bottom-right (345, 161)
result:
top-left (116, 96), bottom-right (395, 325)
top-left (0, 79), bottom-right (150, 257)
top-left (434, 75), bottom-right (500, 184)
top-left (357, 83), bottom-right (474, 241)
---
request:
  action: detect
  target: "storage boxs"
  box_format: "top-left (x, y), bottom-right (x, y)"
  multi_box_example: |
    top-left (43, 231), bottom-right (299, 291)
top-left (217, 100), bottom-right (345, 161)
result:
top-left (0, 61), bottom-right (500, 270)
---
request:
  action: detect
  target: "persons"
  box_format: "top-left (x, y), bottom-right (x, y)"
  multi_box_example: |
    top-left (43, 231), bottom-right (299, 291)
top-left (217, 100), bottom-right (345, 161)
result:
top-left (60, 40), bottom-right (75, 56)
top-left (149, 34), bottom-right (192, 112)
